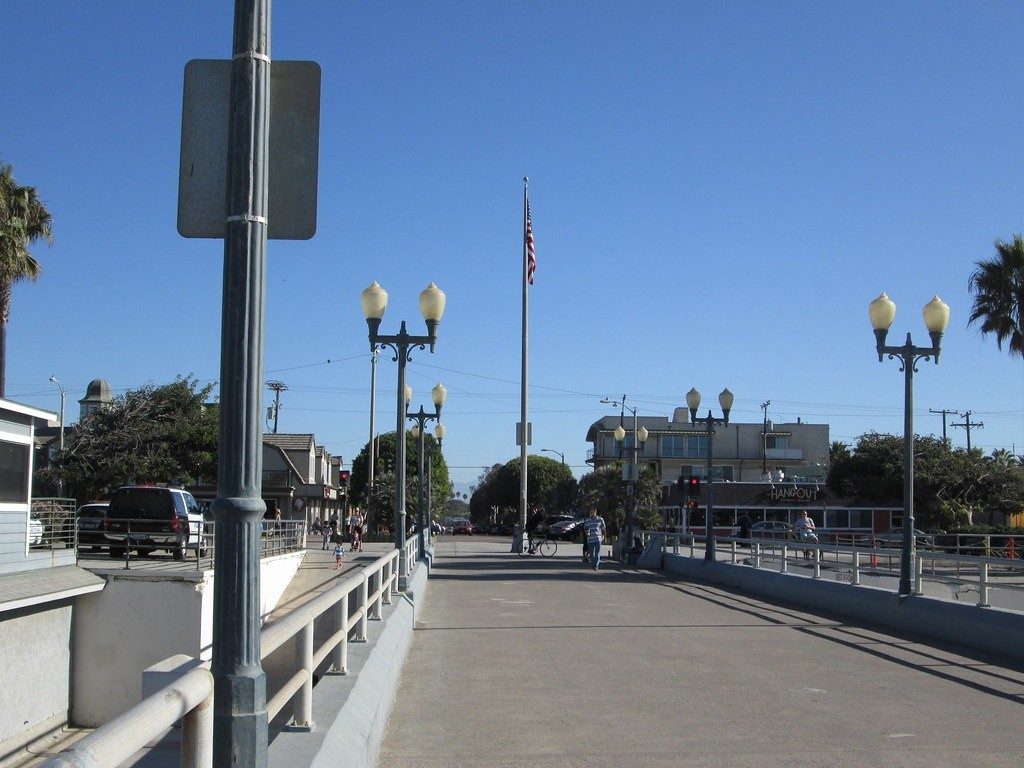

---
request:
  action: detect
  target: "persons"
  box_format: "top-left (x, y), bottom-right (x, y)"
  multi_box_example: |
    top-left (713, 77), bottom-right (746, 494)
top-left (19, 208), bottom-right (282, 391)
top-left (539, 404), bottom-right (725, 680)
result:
top-left (526, 511), bottom-right (543, 554)
top-left (791, 510), bottom-right (818, 553)
top-left (275, 508), bottom-right (281, 535)
top-left (315, 517), bottom-right (324, 535)
top-left (350, 507), bottom-right (363, 551)
top-left (737, 513), bottom-right (752, 566)
top-left (331, 510), bottom-right (339, 534)
top-left (323, 521), bottom-right (332, 550)
top-left (353, 530), bottom-right (360, 546)
top-left (584, 507), bottom-right (606, 571)
top-left (333, 541), bottom-right (344, 570)
top-left (345, 515), bottom-right (351, 534)
top-left (669, 515), bottom-right (675, 533)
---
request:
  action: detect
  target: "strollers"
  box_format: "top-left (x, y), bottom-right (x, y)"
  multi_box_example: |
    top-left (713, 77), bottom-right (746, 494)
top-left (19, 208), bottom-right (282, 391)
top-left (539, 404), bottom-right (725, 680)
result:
top-left (350, 526), bottom-right (362, 552)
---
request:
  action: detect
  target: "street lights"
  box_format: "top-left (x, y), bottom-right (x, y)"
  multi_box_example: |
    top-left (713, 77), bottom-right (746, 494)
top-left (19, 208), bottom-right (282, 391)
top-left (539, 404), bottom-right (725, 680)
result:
top-left (600, 399), bottom-right (640, 466)
top-left (685, 388), bottom-right (734, 562)
top-left (614, 424), bottom-right (649, 553)
top-left (540, 449), bottom-right (565, 514)
top-left (360, 280), bottom-right (448, 593)
top-left (403, 383), bottom-right (447, 559)
top-left (48, 373), bottom-right (66, 498)
top-left (410, 421), bottom-right (446, 544)
top-left (868, 291), bottom-right (950, 596)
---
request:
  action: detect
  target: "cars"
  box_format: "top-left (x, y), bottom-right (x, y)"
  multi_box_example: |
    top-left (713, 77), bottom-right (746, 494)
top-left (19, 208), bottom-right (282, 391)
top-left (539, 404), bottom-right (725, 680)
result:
top-left (450, 519), bottom-right (475, 536)
top-left (859, 526), bottom-right (933, 551)
top-left (445, 515), bottom-right (585, 542)
top-left (60, 501), bottom-right (134, 554)
top-left (750, 520), bottom-right (799, 542)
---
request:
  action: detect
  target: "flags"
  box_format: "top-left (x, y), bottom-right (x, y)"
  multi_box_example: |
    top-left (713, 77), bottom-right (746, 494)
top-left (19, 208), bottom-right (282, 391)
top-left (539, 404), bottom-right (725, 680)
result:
top-left (527, 197), bottom-right (536, 283)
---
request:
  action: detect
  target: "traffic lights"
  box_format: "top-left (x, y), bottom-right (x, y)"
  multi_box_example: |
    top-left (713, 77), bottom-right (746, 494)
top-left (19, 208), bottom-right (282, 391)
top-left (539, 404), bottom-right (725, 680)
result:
top-left (689, 500), bottom-right (698, 508)
top-left (383, 454), bottom-right (392, 474)
top-left (689, 476), bottom-right (700, 495)
top-left (338, 470), bottom-right (350, 488)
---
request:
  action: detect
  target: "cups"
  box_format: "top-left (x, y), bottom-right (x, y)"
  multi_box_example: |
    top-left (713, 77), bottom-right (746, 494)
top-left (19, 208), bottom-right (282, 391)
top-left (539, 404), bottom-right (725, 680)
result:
top-left (587, 530), bottom-right (590, 535)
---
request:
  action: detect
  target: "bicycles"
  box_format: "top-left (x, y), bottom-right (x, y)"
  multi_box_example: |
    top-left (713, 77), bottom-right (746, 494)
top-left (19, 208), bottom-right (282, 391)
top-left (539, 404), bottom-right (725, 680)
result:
top-left (517, 527), bottom-right (558, 558)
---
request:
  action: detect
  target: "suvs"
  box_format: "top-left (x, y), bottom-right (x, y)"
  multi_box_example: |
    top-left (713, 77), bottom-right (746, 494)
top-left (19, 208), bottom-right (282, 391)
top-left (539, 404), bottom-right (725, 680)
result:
top-left (102, 483), bottom-right (210, 561)
